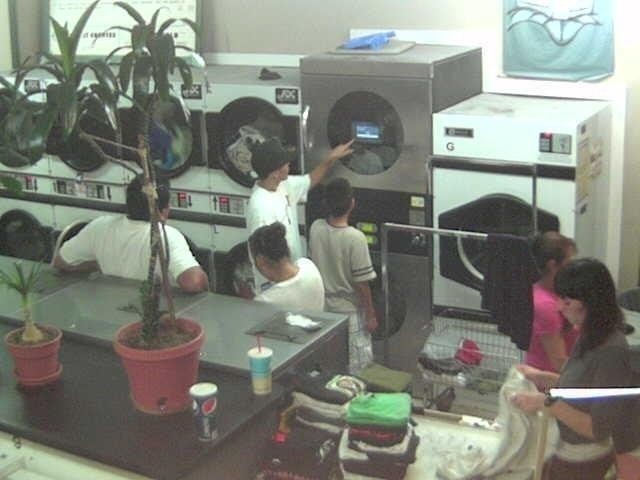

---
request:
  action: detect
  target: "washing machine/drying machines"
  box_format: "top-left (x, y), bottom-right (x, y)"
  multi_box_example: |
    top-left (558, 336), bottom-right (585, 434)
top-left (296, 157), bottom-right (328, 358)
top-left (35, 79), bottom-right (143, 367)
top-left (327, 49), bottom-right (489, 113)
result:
top-left (0, 39), bottom-right (612, 402)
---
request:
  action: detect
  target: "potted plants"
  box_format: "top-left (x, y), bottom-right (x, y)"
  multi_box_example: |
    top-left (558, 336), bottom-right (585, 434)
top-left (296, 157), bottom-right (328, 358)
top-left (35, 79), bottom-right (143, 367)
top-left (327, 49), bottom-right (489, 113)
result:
top-left (17, 0), bottom-right (213, 415)
top-left (0, 66), bottom-right (91, 387)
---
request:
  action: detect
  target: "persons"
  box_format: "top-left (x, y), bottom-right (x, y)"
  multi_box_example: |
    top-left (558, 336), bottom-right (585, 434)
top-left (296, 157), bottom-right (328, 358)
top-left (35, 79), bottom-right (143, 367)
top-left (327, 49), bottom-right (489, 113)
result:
top-left (512, 256), bottom-right (633, 480)
top-left (526, 230), bottom-right (581, 393)
top-left (249, 221), bottom-right (325, 311)
top-left (307, 177), bottom-right (378, 371)
top-left (53, 170), bottom-right (209, 292)
top-left (247, 136), bottom-right (355, 297)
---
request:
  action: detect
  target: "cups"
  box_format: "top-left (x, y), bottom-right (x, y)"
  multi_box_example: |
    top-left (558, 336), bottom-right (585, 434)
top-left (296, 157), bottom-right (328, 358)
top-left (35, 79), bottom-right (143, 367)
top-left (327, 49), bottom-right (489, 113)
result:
top-left (188, 382), bottom-right (220, 441)
top-left (247, 346), bottom-right (273, 394)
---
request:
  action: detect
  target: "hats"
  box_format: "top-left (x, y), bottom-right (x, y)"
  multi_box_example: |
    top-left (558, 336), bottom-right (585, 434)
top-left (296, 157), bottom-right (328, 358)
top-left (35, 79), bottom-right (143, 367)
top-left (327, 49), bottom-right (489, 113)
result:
top-left (250, 137), bottom-right (296, 179)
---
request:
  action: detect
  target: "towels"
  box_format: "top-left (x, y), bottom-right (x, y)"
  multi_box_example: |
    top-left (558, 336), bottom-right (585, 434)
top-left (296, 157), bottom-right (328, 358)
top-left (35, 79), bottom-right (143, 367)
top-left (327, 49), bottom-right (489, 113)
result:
top-left (254, 350), bottom-right (421, 479)
top-left (414, 331), bottom-right (485, 391)
top-left (478, 232), bottom-right (541, 352)
